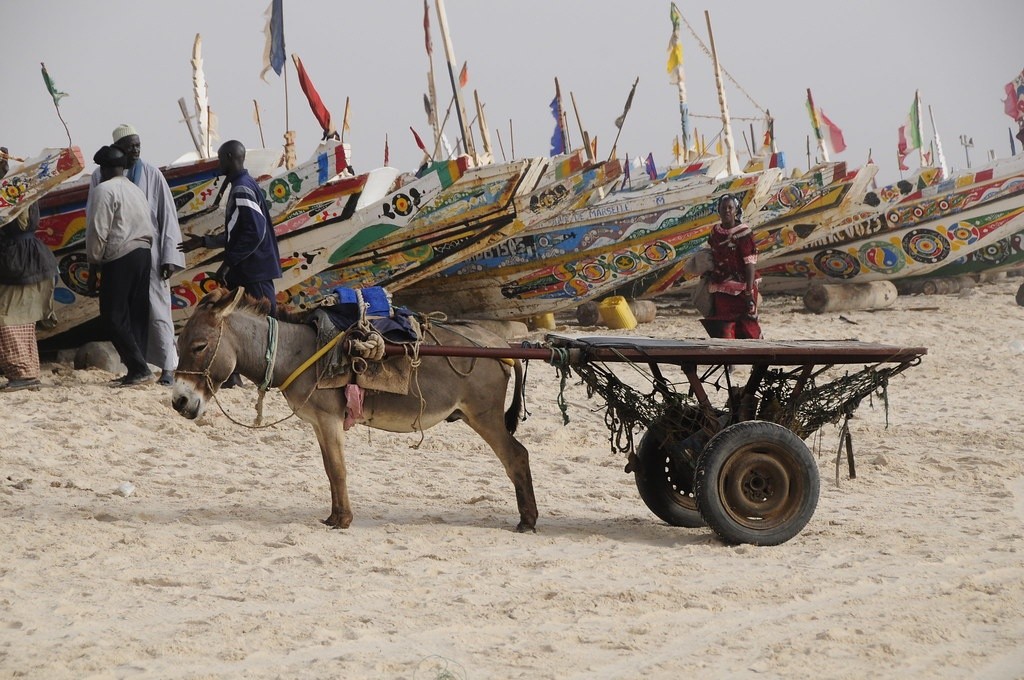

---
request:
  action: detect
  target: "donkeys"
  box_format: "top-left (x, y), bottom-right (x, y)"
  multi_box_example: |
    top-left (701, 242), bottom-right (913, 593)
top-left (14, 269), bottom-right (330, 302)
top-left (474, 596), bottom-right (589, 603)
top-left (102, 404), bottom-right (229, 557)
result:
top-left (171, 286), bottom-right (540, 533)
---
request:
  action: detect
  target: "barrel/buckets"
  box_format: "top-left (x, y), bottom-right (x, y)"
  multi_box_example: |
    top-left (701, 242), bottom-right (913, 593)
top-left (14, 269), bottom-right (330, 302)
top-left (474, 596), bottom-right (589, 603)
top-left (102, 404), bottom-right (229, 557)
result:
top-left (534, 313), bottom-right (556, 332)
top-left (599, 295), bottom-right (637, 331)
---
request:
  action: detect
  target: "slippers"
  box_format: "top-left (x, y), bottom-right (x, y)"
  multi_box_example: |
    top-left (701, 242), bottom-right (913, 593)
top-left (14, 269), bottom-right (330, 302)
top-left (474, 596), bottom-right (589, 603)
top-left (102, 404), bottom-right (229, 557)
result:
top-left (157, 372), bottom-right (174, 385)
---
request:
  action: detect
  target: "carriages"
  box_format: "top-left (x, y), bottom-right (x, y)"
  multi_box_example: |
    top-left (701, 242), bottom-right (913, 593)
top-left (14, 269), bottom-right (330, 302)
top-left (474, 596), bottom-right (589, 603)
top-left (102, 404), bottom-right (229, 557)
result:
top-left (171, 286), bottom-right (929, 547)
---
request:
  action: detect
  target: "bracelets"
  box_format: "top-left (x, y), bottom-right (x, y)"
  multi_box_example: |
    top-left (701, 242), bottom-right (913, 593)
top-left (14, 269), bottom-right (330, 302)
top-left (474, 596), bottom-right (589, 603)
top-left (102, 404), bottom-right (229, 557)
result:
top-left (743, 289), bottom-right (754, 296)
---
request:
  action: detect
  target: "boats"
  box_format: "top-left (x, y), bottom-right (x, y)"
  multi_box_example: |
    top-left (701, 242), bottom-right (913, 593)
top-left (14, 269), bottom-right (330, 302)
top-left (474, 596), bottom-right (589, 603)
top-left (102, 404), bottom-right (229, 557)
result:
top-left (0, 0), bottom-right (1024, 357)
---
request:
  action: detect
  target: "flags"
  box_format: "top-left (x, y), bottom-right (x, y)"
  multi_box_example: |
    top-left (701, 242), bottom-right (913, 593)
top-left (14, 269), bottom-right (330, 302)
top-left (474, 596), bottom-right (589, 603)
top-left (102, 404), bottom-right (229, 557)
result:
top-left (261, 0), bottom-right (1024, 183)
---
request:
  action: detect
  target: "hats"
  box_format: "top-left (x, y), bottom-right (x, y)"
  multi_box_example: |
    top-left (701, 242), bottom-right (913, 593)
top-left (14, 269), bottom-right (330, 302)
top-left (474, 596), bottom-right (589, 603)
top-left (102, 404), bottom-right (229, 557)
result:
top-left (112, 124), bottom-right (136, 144)
top-left (93, 144), bottom-right (127, 166)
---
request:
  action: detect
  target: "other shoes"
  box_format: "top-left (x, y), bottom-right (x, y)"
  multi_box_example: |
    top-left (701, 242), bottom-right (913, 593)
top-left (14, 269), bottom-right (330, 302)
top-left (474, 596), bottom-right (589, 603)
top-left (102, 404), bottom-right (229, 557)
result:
top-left (0, 377), bottom-right (42, 393)
top-left (119, 375), bottom-right (153, 388)
top-left (110, 375), bottom-right (127, 383)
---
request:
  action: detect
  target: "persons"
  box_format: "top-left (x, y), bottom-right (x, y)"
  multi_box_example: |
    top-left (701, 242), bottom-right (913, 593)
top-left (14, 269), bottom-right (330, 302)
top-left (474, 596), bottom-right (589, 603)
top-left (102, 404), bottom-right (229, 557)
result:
top-left (86, 145), bottom-right (159, 387)
top-left (700, 194), bottom-right (764, 373)
top-left (88, 125), bottom-right (186, 385)
top-left (176, 140), bottom-right (282, 388)
top-left (0, 148), bottom-right (60, 392)
